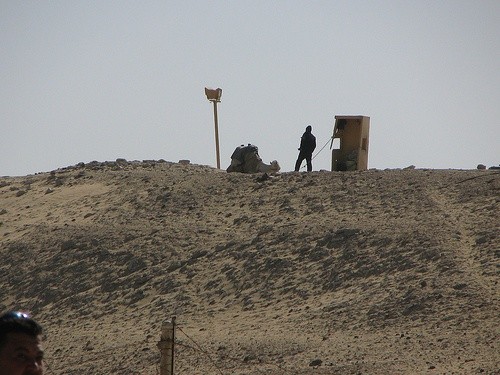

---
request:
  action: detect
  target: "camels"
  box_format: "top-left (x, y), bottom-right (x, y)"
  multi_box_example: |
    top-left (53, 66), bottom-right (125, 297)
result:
top-left (226, 149), bottom-right (281, 174)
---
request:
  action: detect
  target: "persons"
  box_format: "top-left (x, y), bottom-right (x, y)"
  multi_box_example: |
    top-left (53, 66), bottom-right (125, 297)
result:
top-left (1, 311), bottom-right (44, 375)
top-left (294, 125), bottom-right (317, 172)
